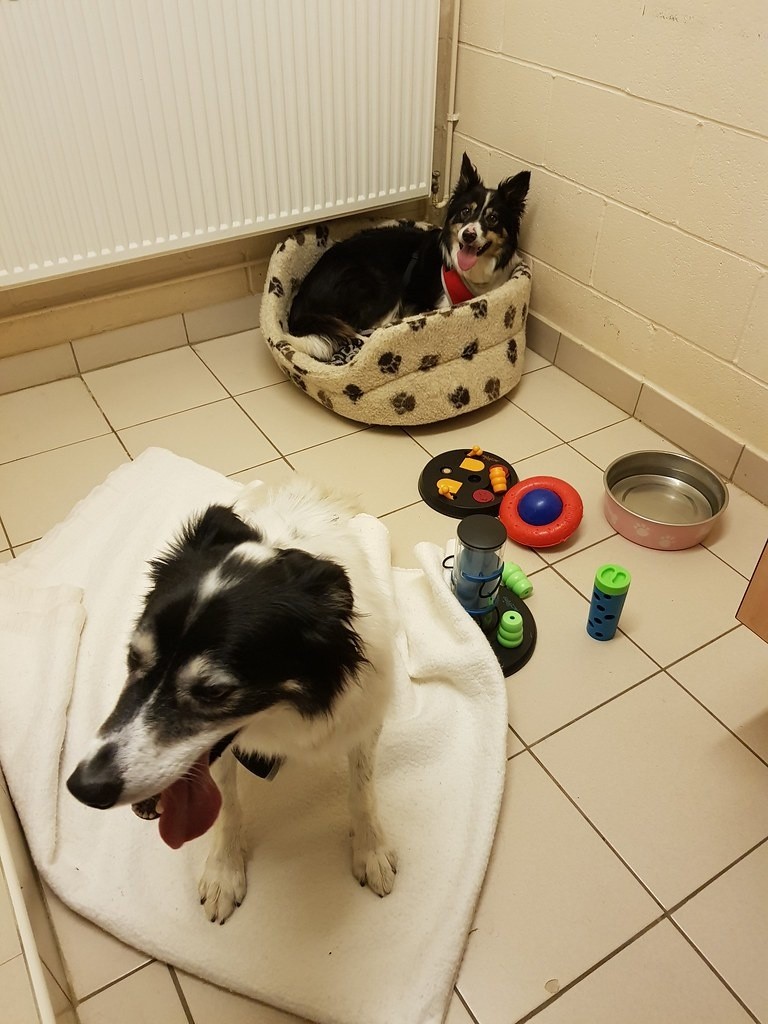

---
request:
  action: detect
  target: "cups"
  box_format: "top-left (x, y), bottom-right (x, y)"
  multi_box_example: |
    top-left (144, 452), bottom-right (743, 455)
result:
top-left (451, 515), bottom-right (507, 614)
top-left (587, 567), bottom-right (630, 643)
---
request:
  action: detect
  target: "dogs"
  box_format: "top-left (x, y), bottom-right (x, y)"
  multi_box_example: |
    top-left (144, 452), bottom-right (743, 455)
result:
top-left (65, 483), bottom-right (398, 927)
top-left (278, 150), bottom-right (533, 361)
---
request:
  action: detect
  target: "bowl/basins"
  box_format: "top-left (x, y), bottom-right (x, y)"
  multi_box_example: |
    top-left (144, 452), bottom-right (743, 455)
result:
top-left (602, 448), bottom-right (730, 551)
